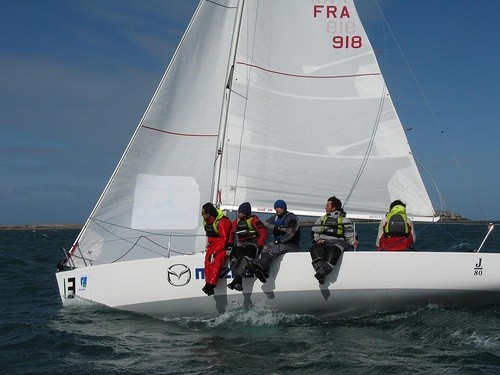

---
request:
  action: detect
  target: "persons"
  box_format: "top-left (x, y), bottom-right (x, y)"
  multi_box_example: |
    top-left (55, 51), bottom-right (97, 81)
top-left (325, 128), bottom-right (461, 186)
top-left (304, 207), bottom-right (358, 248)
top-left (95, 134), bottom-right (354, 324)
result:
top-left (201, 202), bottom-right (232, 296)
top-left (226, 202), bottom-right (268, 291)
top-left (254, 200), bottom-right (301, 283)
top-left (309, 196), bottom-right (358, 285)
top-left (376, 199), bottom-right (416, 251)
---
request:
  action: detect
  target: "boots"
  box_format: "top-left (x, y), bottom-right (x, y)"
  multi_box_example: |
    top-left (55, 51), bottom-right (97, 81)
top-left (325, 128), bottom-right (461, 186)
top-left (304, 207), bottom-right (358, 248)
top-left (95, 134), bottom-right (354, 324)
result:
top-left (312, 257), bottom-right (334, 284)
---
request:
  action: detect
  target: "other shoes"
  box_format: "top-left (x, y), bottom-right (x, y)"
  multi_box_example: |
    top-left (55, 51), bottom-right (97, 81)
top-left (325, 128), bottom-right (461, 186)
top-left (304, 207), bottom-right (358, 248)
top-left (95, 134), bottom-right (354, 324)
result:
top-left (202, 283), bottom-right (215, 296)
top-left (249, 262), bottom-right (260, 270)
top-left (255, 269), bottom-right (266, 283)
top-left (228, 276), bottom-right (243, 291)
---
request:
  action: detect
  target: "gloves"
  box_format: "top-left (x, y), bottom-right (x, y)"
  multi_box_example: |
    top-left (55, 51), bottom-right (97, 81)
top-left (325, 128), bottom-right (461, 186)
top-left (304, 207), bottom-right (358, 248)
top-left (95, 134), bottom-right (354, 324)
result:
top-left (211, 254), bottom-right (216, 264)
top-left (226, 247), bottom-right (232, 256)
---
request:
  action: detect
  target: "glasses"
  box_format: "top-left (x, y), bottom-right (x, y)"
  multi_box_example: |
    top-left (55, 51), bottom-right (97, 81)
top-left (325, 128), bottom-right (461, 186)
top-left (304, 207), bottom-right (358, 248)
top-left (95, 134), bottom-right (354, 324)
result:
top-left (202, 212), bottom-right (205, 214)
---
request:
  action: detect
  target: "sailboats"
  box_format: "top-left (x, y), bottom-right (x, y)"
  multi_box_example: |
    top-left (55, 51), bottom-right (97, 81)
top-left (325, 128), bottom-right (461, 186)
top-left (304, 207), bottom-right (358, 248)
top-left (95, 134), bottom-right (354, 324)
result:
top-left (54, 0), bottom-right (500, 319)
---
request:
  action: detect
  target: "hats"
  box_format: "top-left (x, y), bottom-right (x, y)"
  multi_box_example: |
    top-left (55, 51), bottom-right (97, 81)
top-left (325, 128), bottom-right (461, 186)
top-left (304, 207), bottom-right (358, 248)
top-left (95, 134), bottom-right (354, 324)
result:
top-left (274, 200), bottom-right (287, 211)
top-left (238, 202), bottom-right (251, 215)
